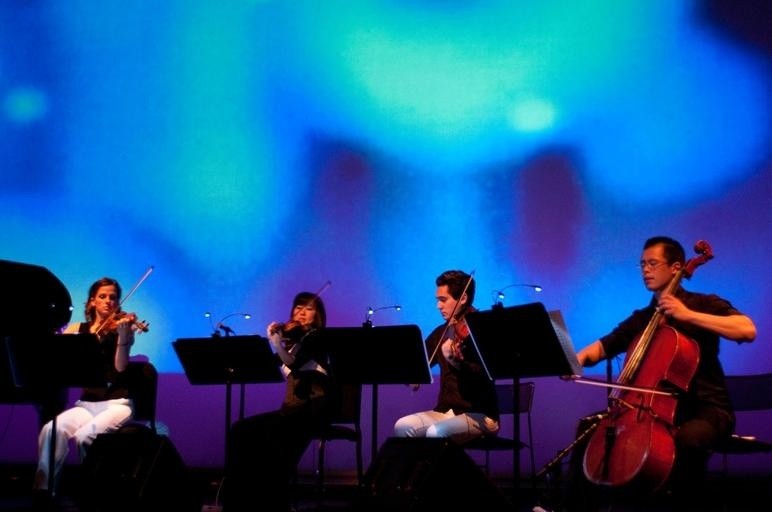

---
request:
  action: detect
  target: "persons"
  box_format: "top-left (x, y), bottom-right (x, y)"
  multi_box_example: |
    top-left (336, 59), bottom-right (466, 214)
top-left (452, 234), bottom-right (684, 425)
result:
top-left (221, 292), bottom-right (347, 512)
top-left (30, 277), bottom-right (141, 492)
top-left (532, 235), bottom-right (759, 512)
top-left (389, 268), bottom-right (504, 449)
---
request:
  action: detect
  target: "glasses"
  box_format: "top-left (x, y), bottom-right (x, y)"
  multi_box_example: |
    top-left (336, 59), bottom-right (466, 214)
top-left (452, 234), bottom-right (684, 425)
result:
top-left (634, 259), bottom-right (670, 271)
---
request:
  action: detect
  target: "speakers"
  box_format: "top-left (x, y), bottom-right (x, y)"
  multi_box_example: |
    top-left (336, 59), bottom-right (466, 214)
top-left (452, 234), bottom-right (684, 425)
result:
top-left (356, 435), bottom-right (482, 512)
top-left (72, 434), bottom-right (204, 511)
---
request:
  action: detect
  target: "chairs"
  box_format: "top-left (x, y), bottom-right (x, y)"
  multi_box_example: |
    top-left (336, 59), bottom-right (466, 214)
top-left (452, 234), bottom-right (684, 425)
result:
top-left (69, 360), bottom-right (158, 458)
top-left (704, 370), bottom-right (772, 479)
top-left (458, 381), bottom-right (535, 479)
top-left (308, 378), bottom-right (364, 491)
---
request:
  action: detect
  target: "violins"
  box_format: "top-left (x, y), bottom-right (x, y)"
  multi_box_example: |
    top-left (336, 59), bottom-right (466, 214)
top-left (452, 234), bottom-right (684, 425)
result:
top-left (96, 311), bottom-right (150, 339)
top-left (269, 318), bottom-right (322, 372)
top-left (447, 303), bottom-right (479, 363)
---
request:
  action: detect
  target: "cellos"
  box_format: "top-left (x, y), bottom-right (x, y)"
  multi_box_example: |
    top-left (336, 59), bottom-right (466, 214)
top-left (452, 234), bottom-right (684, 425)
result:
top-left (580, 238), bottom-right (715, 489)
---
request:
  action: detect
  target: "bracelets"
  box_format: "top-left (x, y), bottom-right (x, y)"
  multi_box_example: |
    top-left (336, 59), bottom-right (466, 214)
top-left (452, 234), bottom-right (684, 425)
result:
top-left (117, 339), bottom-right (131, 346)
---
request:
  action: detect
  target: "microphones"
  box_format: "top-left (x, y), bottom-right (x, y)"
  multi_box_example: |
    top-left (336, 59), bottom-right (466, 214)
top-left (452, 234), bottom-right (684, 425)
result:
top-left (217, 322), bottom-right (234, 334)
